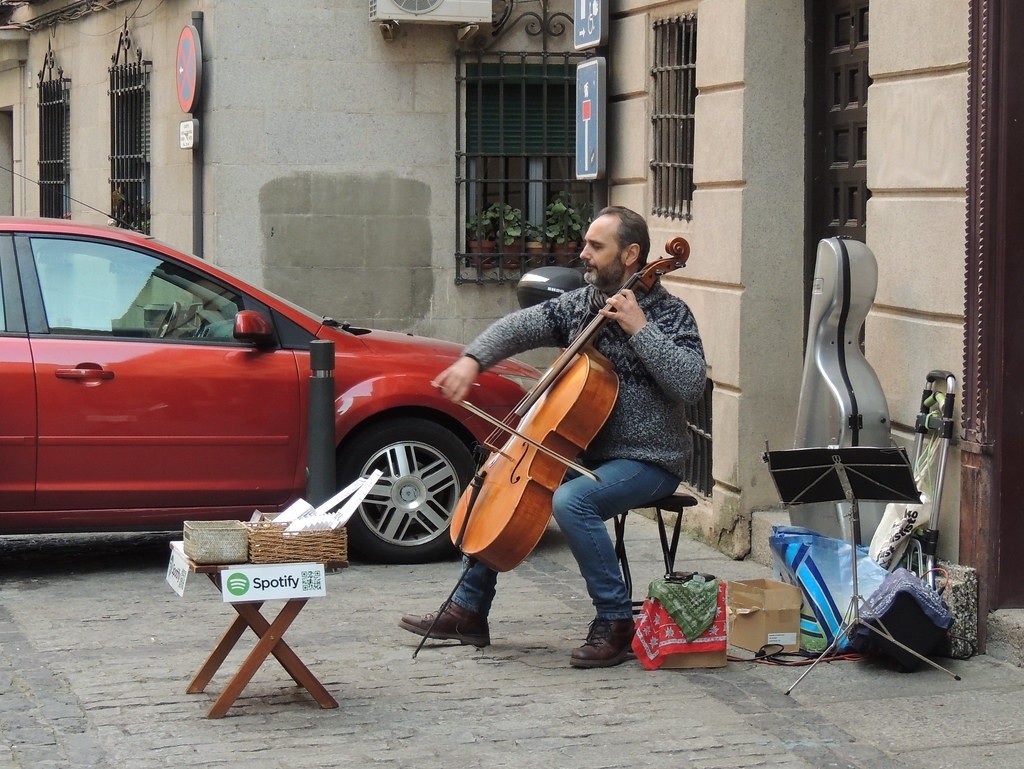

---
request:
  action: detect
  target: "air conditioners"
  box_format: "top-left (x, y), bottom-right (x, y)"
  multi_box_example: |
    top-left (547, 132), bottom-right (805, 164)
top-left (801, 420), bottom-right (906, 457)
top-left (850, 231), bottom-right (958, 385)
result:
top-left (367, 0), bottom-right (494, 28)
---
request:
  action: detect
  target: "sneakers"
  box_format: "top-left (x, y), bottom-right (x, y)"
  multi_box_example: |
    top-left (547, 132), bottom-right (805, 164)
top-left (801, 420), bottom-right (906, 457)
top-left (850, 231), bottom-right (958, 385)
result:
top-left (571, 617), bottom-right (635, 668)
top-left (399, 598), bottom-right (490, 646)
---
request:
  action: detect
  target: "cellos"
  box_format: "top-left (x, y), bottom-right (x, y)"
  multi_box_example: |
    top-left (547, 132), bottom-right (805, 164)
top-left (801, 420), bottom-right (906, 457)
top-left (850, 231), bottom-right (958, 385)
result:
top-left (449, 236), bottom-right (691, 573)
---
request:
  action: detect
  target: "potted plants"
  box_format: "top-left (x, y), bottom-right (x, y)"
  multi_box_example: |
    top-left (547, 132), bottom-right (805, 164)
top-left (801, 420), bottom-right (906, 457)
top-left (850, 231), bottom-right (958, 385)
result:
top-left (466, 190), bottom-right (595, 271)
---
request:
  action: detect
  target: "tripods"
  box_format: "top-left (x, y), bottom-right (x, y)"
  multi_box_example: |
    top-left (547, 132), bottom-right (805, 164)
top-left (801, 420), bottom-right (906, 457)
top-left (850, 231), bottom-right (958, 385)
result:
top-left (757, 439), bottom-right (961, 697)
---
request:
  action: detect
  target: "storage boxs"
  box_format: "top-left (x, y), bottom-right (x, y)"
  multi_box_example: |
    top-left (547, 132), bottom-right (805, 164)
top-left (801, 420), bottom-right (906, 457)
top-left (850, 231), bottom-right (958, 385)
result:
top-left (650, 578), bottom-right (728, 668)
top-left (724, 577), bottom-right (804, 655)
top-left (262, 512), bottom-right (280, 526)
top-left (183, 520), bottom-right (248, 564)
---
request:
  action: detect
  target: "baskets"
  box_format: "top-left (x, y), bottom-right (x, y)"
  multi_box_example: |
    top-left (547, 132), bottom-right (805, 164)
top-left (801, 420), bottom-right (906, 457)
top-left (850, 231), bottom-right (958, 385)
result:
top-left (241, 521), bottom-right (348, 563)
top-left (183, 520), bottom-right (248, 564)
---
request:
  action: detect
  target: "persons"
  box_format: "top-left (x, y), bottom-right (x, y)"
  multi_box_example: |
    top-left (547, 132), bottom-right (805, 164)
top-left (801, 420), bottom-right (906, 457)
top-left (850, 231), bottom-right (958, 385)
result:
top-left (397, 206), bottom-right (707, 667)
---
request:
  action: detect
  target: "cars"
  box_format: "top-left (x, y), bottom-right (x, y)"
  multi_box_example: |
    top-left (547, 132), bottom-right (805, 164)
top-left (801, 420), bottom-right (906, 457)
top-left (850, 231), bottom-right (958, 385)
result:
top-left (0, 214), bottom-right (546, 560)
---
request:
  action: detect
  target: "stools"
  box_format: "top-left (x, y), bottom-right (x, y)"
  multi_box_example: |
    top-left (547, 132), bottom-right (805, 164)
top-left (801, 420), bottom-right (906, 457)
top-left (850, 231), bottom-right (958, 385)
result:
top-left (610, 490), bottom-right (699, 616)
top-left (167, 540), bottom-right (339, 719)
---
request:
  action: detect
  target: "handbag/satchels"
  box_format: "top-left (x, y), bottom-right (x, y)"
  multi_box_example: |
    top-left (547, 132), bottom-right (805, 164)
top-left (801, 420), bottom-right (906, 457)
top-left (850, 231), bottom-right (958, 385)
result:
top-left (769, 523), bottom-right (891, 659)
top-left (868, 435), bottom-right (942, 570)
